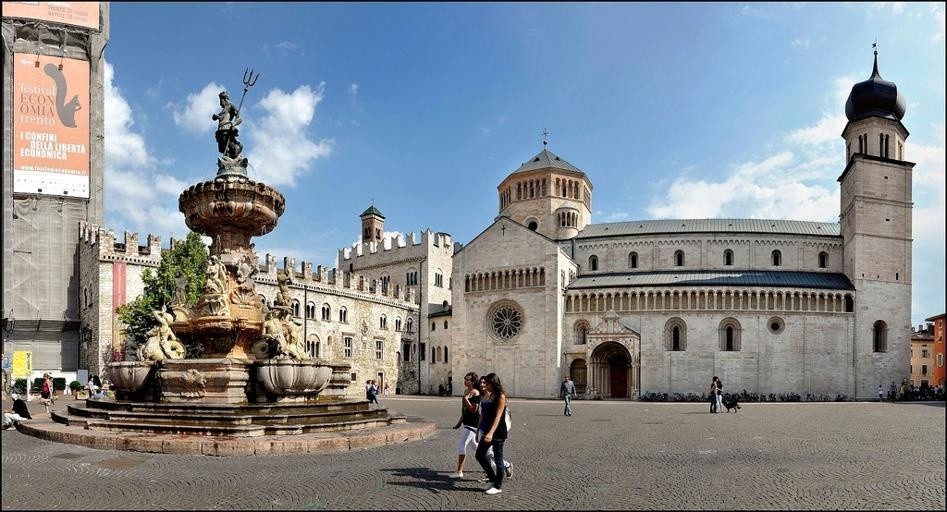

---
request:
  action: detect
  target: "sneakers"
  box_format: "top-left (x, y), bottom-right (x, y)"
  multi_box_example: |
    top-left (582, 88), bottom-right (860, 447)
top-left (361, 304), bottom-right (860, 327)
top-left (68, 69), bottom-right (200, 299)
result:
top-left (485, 486), bottom-right (502, 495)
top-left (6, 427), bottom-right (17, 431)
top-left (506, 463), bottom-right (513, 479)
top-left (564, 413), bottom-right (572, 416)
top-left (478, 476), bottom-right (491, 482)
top-left (448, 471), bottom-right (463, 478)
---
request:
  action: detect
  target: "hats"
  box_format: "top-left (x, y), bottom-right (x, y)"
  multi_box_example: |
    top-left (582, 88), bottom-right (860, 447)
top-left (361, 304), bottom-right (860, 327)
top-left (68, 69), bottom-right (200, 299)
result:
top-left (11, 393), bottom-right (18, 401)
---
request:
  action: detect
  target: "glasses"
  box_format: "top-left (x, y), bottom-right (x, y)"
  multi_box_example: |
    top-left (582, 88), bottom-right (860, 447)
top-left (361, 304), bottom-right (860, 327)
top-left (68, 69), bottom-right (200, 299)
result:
top-left (463, 377), bottom-right (470, 381)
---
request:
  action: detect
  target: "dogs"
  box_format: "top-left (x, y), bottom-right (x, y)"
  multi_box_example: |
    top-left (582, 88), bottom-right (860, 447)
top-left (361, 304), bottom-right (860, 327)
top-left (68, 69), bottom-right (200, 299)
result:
top-left (721, 400), bottom-right (742, 413)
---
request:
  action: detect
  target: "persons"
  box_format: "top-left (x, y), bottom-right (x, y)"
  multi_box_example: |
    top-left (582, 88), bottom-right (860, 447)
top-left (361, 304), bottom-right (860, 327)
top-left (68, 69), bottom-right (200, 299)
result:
top-left (2, 373), bottom-right (110, 431)
top-left (261, 311), bottom-right (286, 347)
top-left (205, 254), bottom-right (227, 294)
top-left (715, 380), bottom-right (725, 413)
top-left (878, 382), bottom-right (943, 402)
top-left (475, 376), bottom-right (513, 481)
top-left (476, 373), bottom-right (508, 495)
top-left (152, 305), bottom-right (177, 341)
top-left (560, 376), bottom-right (577, 417)
top-left (285, 314), bottom-right (303, 345)
top-left (366, 380), bottom-right (372, 399)
top-left (212, 91), bottom-right (242, 168)
top-left (277, 271), bottom-right (293, 300)
top-left (448, 373), bottom-right (479, 479)
top-left (369, 380), bottom-right (378, 404)
top-left (174, 272), bottom-right (188, 303)
top-left (709, 377), bottom-right (718, 413)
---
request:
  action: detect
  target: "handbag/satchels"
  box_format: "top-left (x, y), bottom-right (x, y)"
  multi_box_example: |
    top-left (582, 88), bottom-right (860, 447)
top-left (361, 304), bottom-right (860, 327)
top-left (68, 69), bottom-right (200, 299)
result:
top-left (505, 408), bottom-right (512, 431)
